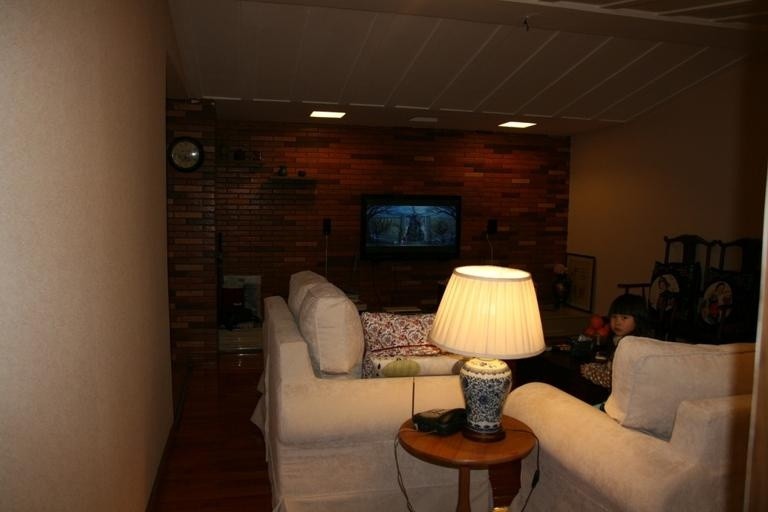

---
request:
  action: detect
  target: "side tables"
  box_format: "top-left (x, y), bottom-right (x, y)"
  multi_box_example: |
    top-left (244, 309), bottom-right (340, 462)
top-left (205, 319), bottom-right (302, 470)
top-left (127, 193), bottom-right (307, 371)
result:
top-left (398, 409), bottom-right (538, 510)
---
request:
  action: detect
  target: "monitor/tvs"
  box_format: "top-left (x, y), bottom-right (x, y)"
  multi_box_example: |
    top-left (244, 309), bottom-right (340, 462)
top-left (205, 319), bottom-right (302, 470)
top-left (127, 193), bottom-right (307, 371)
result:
top-left (358, 192), bottom-right (463, 266)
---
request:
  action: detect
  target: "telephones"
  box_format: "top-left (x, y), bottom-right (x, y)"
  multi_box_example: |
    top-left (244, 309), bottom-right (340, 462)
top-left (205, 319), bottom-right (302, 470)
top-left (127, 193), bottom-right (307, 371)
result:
top-left (413, 408), bottom-right (464, 436)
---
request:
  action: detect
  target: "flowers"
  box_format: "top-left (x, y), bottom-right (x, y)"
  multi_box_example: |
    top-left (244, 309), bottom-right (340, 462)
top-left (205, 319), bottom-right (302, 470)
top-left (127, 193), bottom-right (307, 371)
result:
top-left (551, 264), bottom-right (574, 305)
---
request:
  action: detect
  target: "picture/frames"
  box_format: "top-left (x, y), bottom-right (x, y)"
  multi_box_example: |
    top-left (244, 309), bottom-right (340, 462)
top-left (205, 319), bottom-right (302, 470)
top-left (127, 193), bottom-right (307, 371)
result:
top-left (563, 253), bottom-right (595, 314)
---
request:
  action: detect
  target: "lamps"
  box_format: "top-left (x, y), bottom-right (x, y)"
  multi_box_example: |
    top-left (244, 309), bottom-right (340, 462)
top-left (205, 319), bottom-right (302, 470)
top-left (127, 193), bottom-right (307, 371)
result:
top-left (427, 265), bottom-right (547, 442)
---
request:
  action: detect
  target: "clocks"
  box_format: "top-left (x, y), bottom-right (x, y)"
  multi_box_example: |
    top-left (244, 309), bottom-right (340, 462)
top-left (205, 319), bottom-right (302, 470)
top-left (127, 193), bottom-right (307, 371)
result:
top-left (168, 136), bottom-right (205, 172)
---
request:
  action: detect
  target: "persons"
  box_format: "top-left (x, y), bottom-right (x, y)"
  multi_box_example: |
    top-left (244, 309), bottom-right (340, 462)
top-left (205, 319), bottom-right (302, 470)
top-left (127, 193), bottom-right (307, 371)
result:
top-left (581, 292), bottom-right (658, 401)
top-left (656, 277), bottom-right (672, 311)
top-left (703, 283), bottom-right (727, 323)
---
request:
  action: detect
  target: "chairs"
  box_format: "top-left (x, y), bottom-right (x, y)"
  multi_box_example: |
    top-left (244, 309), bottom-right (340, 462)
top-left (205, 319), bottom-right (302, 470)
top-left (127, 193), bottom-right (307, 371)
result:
top-left (617, 230), bottom-right (762, 342)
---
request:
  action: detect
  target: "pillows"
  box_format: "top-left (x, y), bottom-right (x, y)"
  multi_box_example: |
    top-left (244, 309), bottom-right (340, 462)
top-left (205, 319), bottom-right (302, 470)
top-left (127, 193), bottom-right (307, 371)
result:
top-left (288, 270), bottom-right (462, 378)
top-left (603, 335), bottom-right (755, 440)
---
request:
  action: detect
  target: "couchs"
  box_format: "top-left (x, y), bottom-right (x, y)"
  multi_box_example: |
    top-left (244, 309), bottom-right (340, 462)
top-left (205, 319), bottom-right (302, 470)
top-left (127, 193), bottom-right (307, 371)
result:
top-left (263, 295), bottom-right (490, 512)
top-left (501, 381), bottom-right (756, 512)
